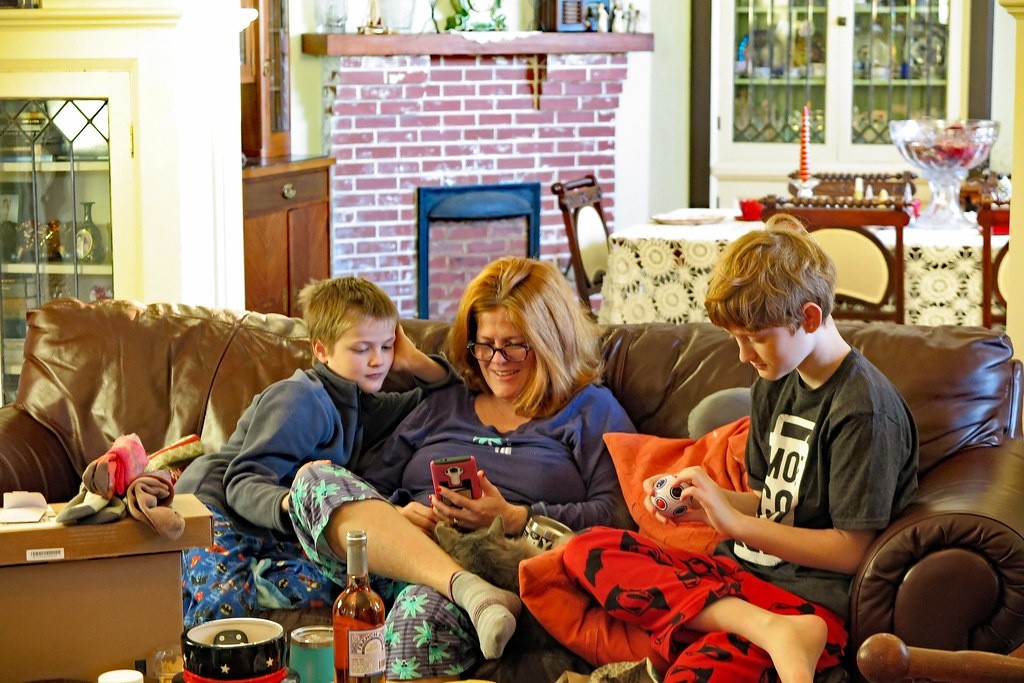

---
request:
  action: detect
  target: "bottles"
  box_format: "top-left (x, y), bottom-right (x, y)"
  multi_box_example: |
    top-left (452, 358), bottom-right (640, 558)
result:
top-left (76, 202), bottom-right (104, 263)
top-left (332, 529), bottom-right (386, 683)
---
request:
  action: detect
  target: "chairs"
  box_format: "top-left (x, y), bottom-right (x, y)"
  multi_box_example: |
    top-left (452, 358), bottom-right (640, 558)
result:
top-left (973, 210), bottom-right (1010, 331)
top-left (552, 174), bottom-right (611, 322)
top-left (762, 208), bottom-right (911, 325)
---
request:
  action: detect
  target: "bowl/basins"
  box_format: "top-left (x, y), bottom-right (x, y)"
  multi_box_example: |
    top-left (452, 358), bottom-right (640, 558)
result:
top-left (740, 200), bottom-right (763, 216)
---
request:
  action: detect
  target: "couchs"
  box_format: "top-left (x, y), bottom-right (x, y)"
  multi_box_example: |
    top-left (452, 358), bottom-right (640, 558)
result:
top-left (0, 299), bottom-right (1023, 683)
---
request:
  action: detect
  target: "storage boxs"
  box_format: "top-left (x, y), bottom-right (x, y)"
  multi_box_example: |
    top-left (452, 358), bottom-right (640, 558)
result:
top-left (1, 550), bottom-right (182, 683)
top-left (0, 492), bottom-right (214, 570)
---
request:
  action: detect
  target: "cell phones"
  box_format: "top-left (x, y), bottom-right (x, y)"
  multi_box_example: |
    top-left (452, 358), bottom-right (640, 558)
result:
top-left (430, 456), bottom-right (482, 509)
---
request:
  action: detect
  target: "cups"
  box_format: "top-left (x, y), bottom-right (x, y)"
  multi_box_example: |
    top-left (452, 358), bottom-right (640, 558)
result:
top-left (180, 617), bottom-right (291, 683)
top-left (289, 625), bottom-right (336, 683)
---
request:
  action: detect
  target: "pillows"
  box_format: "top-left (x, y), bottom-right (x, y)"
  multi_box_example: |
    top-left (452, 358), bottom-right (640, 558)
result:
top-left (604, 417), bottom-right (751, 555)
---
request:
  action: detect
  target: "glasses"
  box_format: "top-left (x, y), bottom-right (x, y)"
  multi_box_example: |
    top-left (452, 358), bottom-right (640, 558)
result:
top-left (466, 338), bottom-right (534, 363)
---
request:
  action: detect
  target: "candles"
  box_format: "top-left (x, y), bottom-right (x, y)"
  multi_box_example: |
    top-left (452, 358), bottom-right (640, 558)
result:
top-left (800, 105), bottom-right (811, 182)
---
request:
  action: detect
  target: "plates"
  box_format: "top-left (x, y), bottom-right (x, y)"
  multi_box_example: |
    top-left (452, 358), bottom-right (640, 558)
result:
top-left (651, 211), bottom-right (728, 225)
top-left (732, 21), bottom-right (948, 83)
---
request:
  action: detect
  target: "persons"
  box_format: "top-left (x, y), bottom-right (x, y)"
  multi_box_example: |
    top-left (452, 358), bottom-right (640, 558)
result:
top-left (287, 256), bottom-right (639, 683)
top-left (562, 213), bottom-right (919, 683)
top-left (174, 277), bottom-right (465, 630)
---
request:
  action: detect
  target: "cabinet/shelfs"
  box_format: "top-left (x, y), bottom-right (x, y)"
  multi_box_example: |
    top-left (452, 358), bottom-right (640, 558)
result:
top-left (0, 60), bottom-right (182, 406)
top-left (242, 153), bottom-right (336, 320)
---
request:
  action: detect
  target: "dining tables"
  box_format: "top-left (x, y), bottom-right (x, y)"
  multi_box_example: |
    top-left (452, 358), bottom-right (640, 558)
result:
top-left (605, 206), bottom-right (1010, 325)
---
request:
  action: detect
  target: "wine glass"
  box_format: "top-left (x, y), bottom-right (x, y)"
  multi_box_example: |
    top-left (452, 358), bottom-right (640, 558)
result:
top-left (887, 117), bottom-right (1000, 229)
